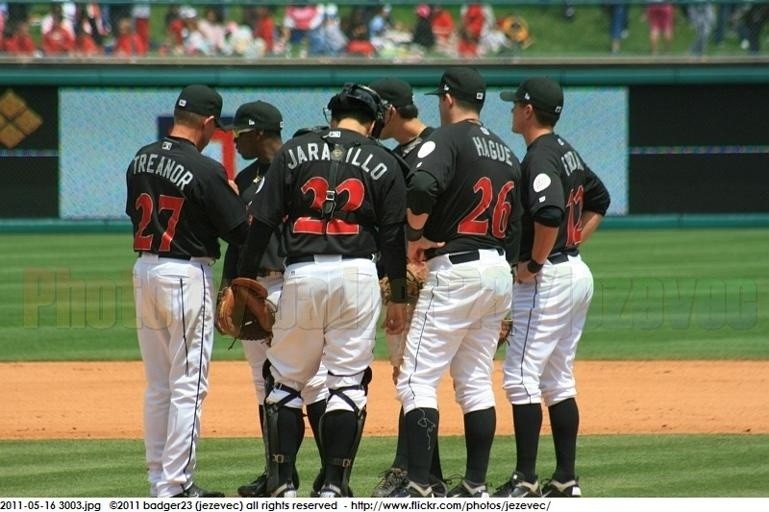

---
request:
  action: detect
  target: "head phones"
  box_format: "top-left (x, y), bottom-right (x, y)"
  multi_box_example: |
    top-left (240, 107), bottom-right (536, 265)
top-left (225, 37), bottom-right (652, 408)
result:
top-left (326, 82), bottom-right (385, 138)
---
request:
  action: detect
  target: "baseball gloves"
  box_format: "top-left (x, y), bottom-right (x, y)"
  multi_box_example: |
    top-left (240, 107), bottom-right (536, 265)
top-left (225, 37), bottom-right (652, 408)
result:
top-left (219, 276), bottom-right (276, 350)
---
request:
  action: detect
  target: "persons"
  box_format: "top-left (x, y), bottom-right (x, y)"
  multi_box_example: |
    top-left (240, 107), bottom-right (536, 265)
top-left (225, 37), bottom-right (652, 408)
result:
top-left (216, 101), bottom-right (286, 498)
top-left (711, 1), bottom-right (734, 46)
top-left (125, 84), bottom-right (251, 498)
top-left (492, 78), bottom-right (611, 497)
top-left (607, 4), bottom-right (630, 56)
top-left (686, 0), bottom-right (716, 54)
top-left (368, 78), bottom-right (438, 498)
top-left (1, 0), bottom-right (530, 60)
top-left (235, 82), bottom-right (407, 497)
top-left (739, 0), bottom-right (768, 53)
top-left (641, 3), bottom-right (675, 58)
top-left (385, 65), bottom-right (522, 498)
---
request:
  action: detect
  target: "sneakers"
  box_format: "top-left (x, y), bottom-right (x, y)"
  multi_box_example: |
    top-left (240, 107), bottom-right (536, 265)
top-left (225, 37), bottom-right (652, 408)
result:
top-left (237, 470), bottom-right (306, 496)
top-left (264, 479), bottom-right (297, 496)
top-left (491, 472), bottom-right (541, 498)
top-left (374, 468), bottom-right (407, 497)
top-left (317, 483), bottom-right (367, 497)
top-left (429, 476), bottom-right (447, 498)
top-left (388, 478), bottom-right (436, 497)
top-left (446, 482), bottom-right (488, 499)
top-left (540, 481), bottom-right (580, 498)
top-left (307, 469), bottom-right (355, 497)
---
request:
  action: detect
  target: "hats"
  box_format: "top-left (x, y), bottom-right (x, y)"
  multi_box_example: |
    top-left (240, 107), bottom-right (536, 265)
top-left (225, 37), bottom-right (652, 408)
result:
top-left (501, 77), bottom-right (564, 113)
top-left (370, 78), bottom-right (413, 107)
top-left (327, 84), bottom-right (382, 118)
top-left (228, 99), bottom-right (284, 131)
top-left (176, 85), bottom-right (227, 131)
top-left (424, 66), bottom-right (485, 104)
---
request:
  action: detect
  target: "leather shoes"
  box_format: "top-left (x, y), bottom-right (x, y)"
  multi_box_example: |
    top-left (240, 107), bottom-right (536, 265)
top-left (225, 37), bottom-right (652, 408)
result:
top-left (173, 483), bottom-right (224, 497)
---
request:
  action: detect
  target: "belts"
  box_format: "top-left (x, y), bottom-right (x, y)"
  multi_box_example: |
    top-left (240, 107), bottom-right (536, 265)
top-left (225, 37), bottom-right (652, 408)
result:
top-left (427, 249), bottom-right (506, 267)
top-left (136, 250), bottom-right (214, 264)
top-left (257, 269), bottom-right (284, 277)
top-left (512, 247), bottom-right (579, 278)
top-left (283, 255), bottom-right (377, 262)
